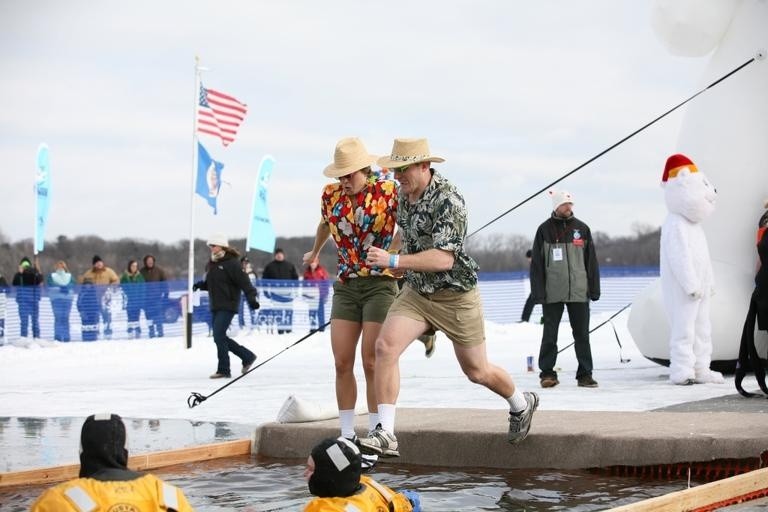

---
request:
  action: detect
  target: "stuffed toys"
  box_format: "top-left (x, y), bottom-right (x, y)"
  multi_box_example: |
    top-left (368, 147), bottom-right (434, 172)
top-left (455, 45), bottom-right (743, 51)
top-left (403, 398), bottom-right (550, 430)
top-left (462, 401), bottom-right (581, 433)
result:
top-left (658, 151), bottom-right (728, 386)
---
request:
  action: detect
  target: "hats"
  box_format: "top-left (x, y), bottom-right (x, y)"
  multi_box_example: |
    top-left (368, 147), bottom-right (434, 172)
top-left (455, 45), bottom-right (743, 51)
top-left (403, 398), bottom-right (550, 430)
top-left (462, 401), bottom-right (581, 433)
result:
top-left (20, 257), bottom-right (32, 267)
top-left (547, 189), bottom-right (575, 212)
top-left (92, 255), bottom-right (102, 265)
top-left (79, 414), bottom-right (147, 482)
top-left (240, 256), bottom-right (249, 263)
top-left (375, 136), bottom-right (445, 168)
top-left (308, 436), bottom-right (361, 497)
top-left (323, 137), bottom-right (379, 179)
top-left (83, 278), bottom-right (94, 284)
top-left (206, 232), bottom-right (230, 249)
top-left (273, 247), bottom-right (284, 255)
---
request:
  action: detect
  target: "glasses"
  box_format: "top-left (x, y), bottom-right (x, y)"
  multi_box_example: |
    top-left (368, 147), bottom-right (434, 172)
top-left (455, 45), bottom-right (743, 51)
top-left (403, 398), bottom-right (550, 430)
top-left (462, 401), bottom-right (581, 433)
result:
top-left (388, 165), bottom-right (410, 174)
top-left (339, 172), bottom-right (356, 180)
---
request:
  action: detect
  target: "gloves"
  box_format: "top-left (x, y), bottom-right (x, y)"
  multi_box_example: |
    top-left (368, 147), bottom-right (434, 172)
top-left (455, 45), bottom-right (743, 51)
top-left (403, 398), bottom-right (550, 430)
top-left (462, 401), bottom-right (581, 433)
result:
top-left (192, 281), bottom-right (205, 292)
top-left (250, 301), bottom-right (260, 310)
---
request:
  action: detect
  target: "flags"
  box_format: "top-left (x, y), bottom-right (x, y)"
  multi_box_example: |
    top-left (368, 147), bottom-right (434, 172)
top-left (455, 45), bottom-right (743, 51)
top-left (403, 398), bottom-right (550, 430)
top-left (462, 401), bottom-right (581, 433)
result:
top-left (194, 72), bottom-right (250, 215)
top-left (37, 149), bottom-right (56, 257)
top-left (247, 157), bottom-right (280, 261)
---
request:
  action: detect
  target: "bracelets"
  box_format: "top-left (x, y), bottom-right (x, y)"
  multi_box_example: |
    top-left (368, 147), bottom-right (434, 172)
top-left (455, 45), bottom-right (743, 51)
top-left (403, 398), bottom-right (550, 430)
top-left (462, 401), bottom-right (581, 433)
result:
top-left (388, 251), bottom-right (402, 270)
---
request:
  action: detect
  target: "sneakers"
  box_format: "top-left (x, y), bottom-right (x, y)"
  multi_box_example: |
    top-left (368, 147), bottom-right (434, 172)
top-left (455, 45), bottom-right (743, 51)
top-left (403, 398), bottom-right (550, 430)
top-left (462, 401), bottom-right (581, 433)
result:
top-left (507, 392), bottom-right (540, 444)
top-left (241, 354), bottom-right (256, 373)
top-left (540, 373), bottom-right (558, 387)
top-left (425, 334), bottom-right (436, 357)
top-left (578, 375), bottom-right (598, 387)
top-left (211, 373), bottom-right (231, 378)
top-left (347, 422), bottom-right (400, 469)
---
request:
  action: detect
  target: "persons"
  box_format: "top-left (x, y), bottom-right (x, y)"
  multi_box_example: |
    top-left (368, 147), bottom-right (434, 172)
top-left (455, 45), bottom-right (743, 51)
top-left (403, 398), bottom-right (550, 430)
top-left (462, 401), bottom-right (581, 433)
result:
top-left (42, 260), bottom-right (76, 343)
top-left (750, 199), bottom-right (768, 374)
top-left (353, 136), bottom-right (538, 456)
top-left (11, 259), bottom-right (41, 339)
top-left (28, 412), bottom-right (199, 511)
top-left (78, 257), bottom-right (122, 342)
top-left (239, 254), bottom-right (258, 328)
top-left (516, 250), bottom-right (538, 324)
top-left (119, 260), bottom-right (148, 339)
top-left (188, 229), bottom-right (261, 380)
top-left (78, 278), bottom-right (103, 343)
top-left (297, 136), bottom-right (404, 467)
top-left (0, 272), bottom-right (10, 344)
top-left (261, 248), bottom-right (298, 334)
top-left (296, 436), bottom-right (421, 512)
top-left (529, 186), bottom-right (604, 391)
top-left (139, 253), bottom-right (170, 338)
top-left (299, 254), bottom-right (331, 333)
